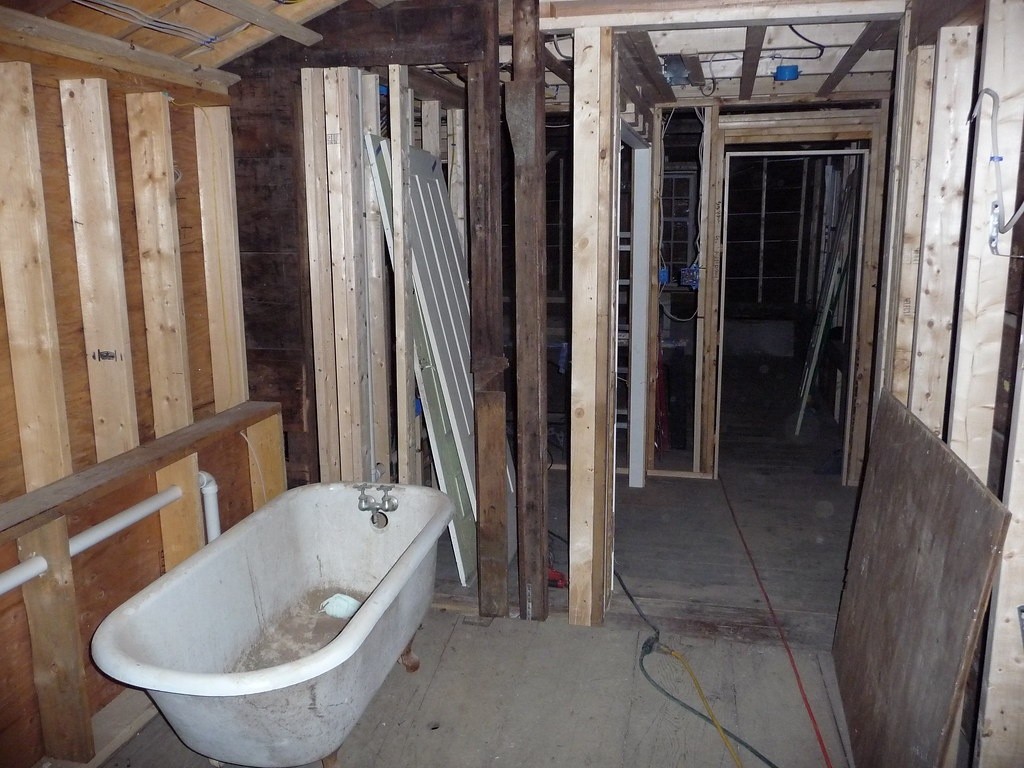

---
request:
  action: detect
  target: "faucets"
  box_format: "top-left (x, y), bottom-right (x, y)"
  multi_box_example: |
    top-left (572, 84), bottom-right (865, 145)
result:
top-left (352, 483), bottom-right (399, 523)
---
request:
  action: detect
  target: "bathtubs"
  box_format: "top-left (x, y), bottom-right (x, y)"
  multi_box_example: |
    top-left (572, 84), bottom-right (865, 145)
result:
top-left (91, 480), bottom-right (457, 767)
